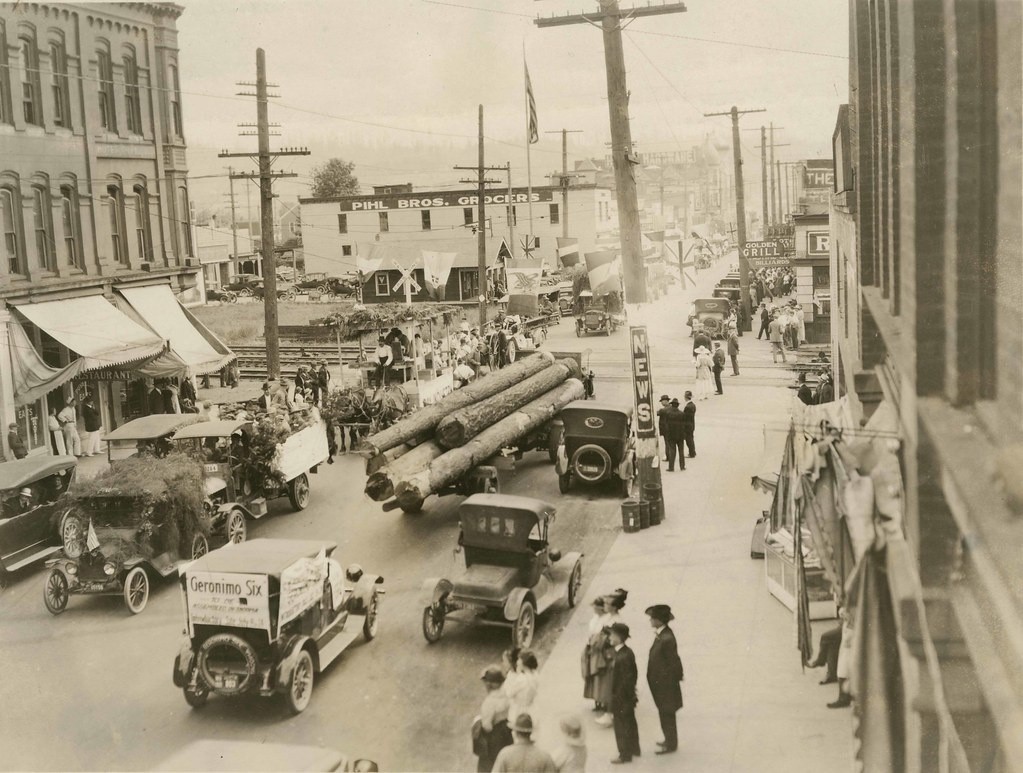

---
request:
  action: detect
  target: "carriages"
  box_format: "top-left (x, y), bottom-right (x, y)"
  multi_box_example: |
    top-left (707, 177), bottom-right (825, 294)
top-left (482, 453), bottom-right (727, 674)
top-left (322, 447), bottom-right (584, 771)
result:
top-left (320, 365), bottom-right (452, 453)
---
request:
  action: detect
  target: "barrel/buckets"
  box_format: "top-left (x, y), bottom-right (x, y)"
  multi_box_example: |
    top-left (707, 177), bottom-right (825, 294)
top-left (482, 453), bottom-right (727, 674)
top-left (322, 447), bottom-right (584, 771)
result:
top-left (621, 482), bottom-right (662, 532)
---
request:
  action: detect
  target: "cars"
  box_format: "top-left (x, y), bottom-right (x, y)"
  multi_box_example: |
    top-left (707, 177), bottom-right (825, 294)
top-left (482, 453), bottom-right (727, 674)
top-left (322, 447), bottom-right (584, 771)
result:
top-left (694, 275), bottom-right (742, 336)
top-left (421, 494), bottom-right (584, 653)
top-left (558, 402), bottom-right (633, 496)
top-left (1, 454), bottom-right (86, 592)
top-left (173, 536), bottom-right (388, 714)
top-left (572, 290), bottom-right (628, 340)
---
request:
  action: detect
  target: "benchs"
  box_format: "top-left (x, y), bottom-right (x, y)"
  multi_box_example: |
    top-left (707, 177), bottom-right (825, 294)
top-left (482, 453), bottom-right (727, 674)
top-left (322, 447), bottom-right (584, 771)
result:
top-left (348, 362), bottom-right (412, 388)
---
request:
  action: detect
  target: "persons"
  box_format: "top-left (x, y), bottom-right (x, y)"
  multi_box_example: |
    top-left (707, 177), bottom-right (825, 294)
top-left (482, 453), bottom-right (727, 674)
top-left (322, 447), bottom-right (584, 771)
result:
top-left (294, 360), bottom-right (331, 407)
top-left (204, 377), bottom-right (320, 459)
top-left (149, 378), bottom-right (214, 414)
top-left (374, 336), bottom-right (393, 386)
top-left (689, 266), bottom-right (834, 405)
top-left (580, 589), bottom-right (641, 762)
top-left (451, 295), bottom-right (553, 385)
top-left (806, 628), bottom-right (850, 708)
top-left (9, 424), bottom-right (28, 459)
top-left (433, 340), bottom-right (445, 369)
top-left (387, 328), bottom-right (410, 356)
top-left (81, 398), bottom-right (105, 457)
top-left (657, 391), bottom-right (696, 471)
top-left (58, 397), bottom-right (85, 457)
top-left (475, 648), bottom-right (587, 773)
top-left (411, 334), bottom-right (425, 357)
top-left (49, 408), bottom-right (66, 456)
top-left (645, 605), bottom-right (684, 754)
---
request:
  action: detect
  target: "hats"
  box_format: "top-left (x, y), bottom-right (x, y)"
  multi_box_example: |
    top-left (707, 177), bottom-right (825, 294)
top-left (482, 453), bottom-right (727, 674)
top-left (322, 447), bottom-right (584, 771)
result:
top-left (797, 373), bottom-right (829, 383)
top-left (507, 713), bottom-right (535, 732)
top-left (479, 668), bottom-right (505, 683)
top-left (154, 379), bottom-right (163, 385)
top-left (694, 345), bottom-right (709, 354)
top-left (377, 328), bottom-right (401, 342)
top-left (84, 396), bottom-right (95, 403)
top-left (9, 423), bottom-right (19, 428)
top-left (262, 360), bottom-right (328, 396)
top-left (758, 302), bottom-right (800, 317)
top-left (660, 391), bottom-right (692, 404)
top-left (66, 397), bottom-right (74, 405)
top-left (20, 488), bottom-right (32, 498)
top-left (555, 715), bottom-right (587, 747)
top-left (590, 589), bottom-right (675, 637)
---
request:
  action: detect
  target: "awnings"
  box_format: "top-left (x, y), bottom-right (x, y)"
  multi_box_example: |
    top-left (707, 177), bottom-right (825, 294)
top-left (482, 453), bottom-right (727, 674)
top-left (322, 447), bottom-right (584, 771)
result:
top-left (120, 285), bottom-right (236, 379)
top-left (14, 295), bottom-right (170, 404)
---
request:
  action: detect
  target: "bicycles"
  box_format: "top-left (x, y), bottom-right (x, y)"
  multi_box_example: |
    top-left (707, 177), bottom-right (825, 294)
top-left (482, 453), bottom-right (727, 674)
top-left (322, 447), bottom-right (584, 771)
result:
top-left (220, 289), bottom-right (238, 304)
top-left (241, 286), bottom-right (261, 302)
top-left (317, 281), bottom-right (360, 302)
top-left (278, 289), bottom-right (298, 302)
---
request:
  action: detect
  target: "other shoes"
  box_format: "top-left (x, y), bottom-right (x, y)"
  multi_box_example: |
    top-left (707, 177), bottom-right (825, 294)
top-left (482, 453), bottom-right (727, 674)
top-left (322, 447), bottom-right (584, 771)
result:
top-left (661, 455), bottom-right (695, 471)
top-left (656, 746), bottom-right (676, 754)
top-left (713, 374), bottom-right (739, 395)
top-left (593, 705), bottom-right (640, 764)
top-left (807, 657), bottom-right (850, 708)
top-left (75, 451), bottom-right (104, 457)
top-left (656, 742), bottom-right (667, 747)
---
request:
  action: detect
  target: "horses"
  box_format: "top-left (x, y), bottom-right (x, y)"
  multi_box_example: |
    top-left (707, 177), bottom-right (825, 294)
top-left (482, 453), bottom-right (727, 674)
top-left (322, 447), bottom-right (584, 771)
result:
top-left (335, 385), bottom-right (409, 456)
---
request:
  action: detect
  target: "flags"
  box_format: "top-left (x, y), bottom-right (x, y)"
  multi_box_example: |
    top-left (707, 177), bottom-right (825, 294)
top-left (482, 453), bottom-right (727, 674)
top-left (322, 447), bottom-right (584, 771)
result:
top-left (527, 67), bottom-right (539, 144)
top-left (520, 233), bottom-right (535, 260)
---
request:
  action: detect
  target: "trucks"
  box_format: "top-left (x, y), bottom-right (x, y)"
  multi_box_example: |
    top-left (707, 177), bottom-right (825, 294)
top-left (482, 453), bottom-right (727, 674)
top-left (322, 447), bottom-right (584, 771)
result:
top-left (368, 351), bottom-right (593, 518)
top-left (46, 414), bottom-right (333, 617)
